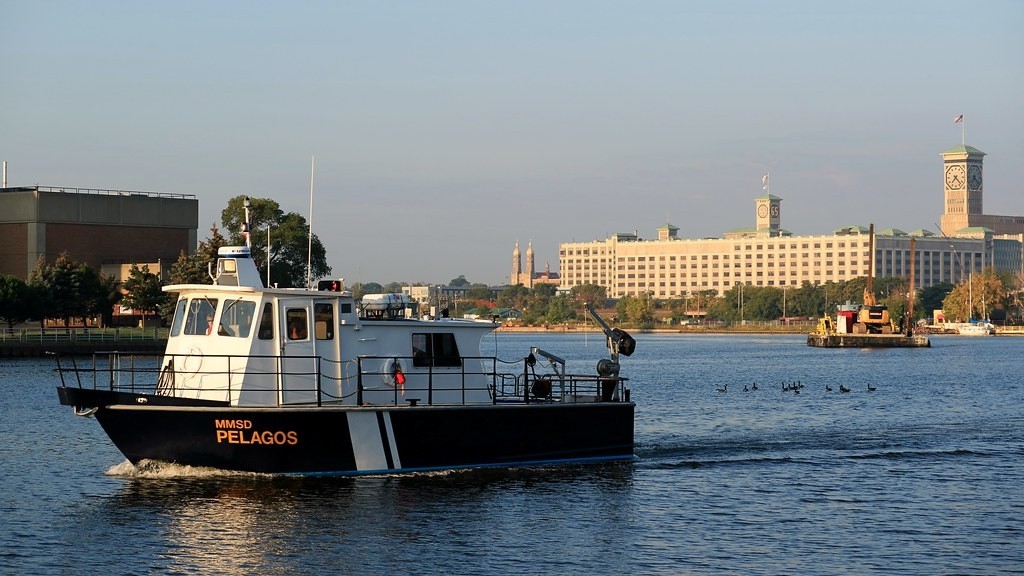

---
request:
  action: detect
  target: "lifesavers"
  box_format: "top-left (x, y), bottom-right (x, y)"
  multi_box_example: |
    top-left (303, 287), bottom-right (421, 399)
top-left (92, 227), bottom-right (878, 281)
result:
top-left (179, 345), bottom-right (204, 378)
top-left (383, 354), bottom-right (408, 388)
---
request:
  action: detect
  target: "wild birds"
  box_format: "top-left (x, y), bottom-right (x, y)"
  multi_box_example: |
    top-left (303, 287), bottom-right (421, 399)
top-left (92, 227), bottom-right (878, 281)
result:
top-left (742, 381), bottom-right (759, 392)
top-left (781, 380), bottom-right (806, 396)
top-left (867, 382), bottom-right (877, 391)
top-left (716, 383), bottom-right (728, 393)
top-left (839, 384), bottom-right (852, 393)
top-left (825, 383), bottom-right (832, 392)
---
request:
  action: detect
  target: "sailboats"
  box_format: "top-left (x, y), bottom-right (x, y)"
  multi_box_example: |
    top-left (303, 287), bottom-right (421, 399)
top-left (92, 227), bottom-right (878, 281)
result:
top-left (955, 273), bottom-right (996, 337)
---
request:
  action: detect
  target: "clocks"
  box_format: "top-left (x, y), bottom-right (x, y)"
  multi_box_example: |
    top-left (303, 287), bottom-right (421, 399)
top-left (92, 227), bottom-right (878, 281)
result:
top-left (945, 164), bottom-right (965, 191)
top-left (967, 165), bottom-right (983, 191)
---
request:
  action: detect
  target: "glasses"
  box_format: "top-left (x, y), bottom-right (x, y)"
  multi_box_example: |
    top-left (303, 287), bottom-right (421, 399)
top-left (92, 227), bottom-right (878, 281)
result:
top-left (207, 319), bottom-right (214, 323)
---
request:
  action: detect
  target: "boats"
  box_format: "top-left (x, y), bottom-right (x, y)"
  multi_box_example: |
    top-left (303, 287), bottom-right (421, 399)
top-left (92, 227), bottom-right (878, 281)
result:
top-left (45, 156), bottom-right (638, 481)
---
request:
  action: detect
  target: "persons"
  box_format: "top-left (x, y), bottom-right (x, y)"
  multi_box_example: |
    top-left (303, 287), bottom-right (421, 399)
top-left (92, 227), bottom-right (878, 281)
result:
top-left (205, 314), bottom-right (214, 335)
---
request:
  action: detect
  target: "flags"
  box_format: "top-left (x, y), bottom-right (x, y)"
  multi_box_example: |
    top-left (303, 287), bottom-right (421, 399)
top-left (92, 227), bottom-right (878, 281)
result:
top-left (760, 172), bottom-right (769, 183)
top-left (953, 114), bottom-right (965, 123)
